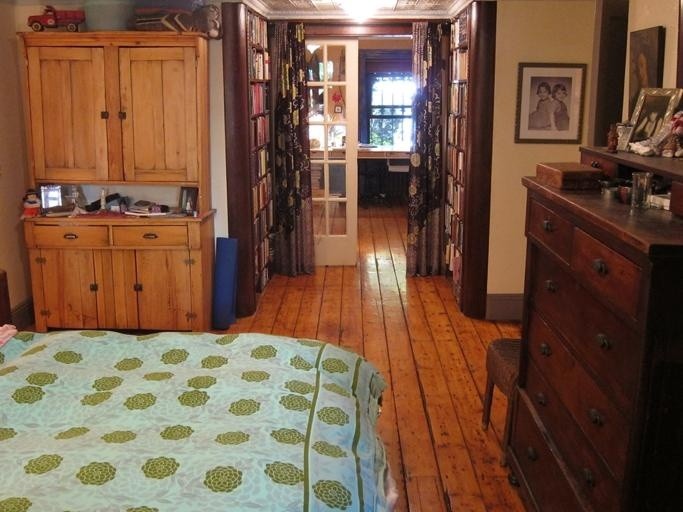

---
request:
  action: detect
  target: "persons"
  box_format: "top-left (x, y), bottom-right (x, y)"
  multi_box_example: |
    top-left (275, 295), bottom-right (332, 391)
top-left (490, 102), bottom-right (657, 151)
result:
top-left (529, 82), bottom-right (569, 131)
top-left (633, 106), bottom-right (664, 138)
top-left (632, 46), bottom-right (653, 113)
top-left (21, 188), bottom-right (41, 220)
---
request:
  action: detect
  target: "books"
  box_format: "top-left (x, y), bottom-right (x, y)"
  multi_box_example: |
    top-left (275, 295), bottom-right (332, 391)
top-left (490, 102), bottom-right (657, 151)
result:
top-left (124, 211), bottom-right (175, 216)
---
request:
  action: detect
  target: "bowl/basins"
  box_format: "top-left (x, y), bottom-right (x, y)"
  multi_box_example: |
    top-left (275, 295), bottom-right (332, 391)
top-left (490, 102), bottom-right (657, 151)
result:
top-left (617, 185), bottom-right (630, 202)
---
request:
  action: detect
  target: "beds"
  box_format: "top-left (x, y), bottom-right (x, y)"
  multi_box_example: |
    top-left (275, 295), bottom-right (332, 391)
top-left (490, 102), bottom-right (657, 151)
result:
top-left (0, 267), bottom-right (401, 512)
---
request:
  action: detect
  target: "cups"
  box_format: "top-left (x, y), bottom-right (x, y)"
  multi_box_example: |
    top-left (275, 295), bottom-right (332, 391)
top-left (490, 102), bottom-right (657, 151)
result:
top-left (629, 171), bottom-right (655, 210)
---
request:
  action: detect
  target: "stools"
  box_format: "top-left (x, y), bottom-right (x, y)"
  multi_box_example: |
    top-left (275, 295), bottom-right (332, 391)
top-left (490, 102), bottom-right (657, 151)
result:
top-left (386, 163), bottom-right (411, 212)
top-left (481, 334), bottom-right (524, 459)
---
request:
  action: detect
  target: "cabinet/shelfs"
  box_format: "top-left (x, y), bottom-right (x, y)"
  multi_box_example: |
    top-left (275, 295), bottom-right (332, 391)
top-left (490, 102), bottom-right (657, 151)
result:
top-left (438, 2), bottom-right (480, 315)
top-left (14, 26), bottom-right (212, 186)
top-left (511, 172), bottom-right (649, 511)
top-left (23, 208), bottom-right (219, 331)
top-left (244, 7), bottom-right (275, 306)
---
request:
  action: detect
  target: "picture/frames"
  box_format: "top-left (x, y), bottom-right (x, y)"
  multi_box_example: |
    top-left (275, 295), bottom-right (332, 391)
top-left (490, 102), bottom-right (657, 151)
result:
top-left (624, 85), bottom-right (682, 142)
top-left (512, 62), bottom-right (587, 145)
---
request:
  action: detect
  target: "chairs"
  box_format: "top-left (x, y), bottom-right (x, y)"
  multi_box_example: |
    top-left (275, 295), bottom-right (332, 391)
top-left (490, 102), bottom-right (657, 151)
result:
top-left (348, 164), bottom-right (385, 209)
